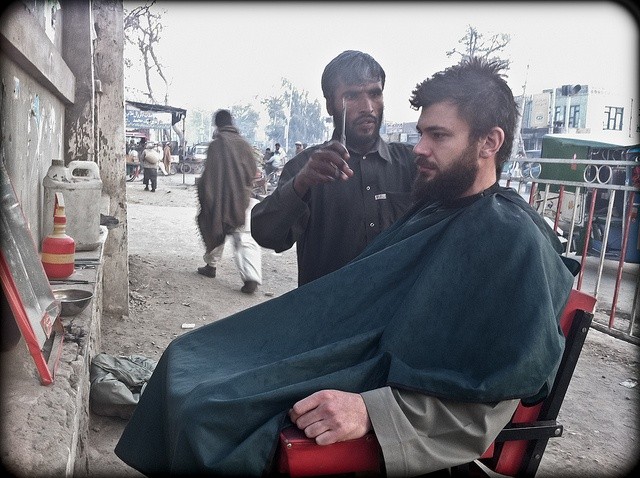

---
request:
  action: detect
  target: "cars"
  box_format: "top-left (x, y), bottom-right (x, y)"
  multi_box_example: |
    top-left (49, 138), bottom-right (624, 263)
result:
top-left (190, 144), bottom-right (209, 161)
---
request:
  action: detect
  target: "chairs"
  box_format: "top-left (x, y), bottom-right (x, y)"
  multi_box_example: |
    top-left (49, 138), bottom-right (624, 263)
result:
top-left (273, 285), bottom-right (596, 478)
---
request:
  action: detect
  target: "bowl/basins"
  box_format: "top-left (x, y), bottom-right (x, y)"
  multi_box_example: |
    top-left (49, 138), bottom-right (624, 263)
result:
top-left (51, 289), bottom-right (96, 316)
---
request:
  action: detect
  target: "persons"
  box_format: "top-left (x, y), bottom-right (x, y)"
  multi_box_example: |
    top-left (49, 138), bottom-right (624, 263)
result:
top-left (114, 55), bottom-right (576, 477)
top-left (263, 148), bottom-right (275, 175)
top-left (153, 140), bottom-right (168, 176)
top-left (250, 49), bottom-right (416, 288)
top-left (138, 141), bottom-right (158, 192)
top-left (194, 110), bottom-right (263, 294)
top-left (266, 150), bottom-right (281, 173)
top-left (130, 145), bottom-right (140, 178)
top-left (164, 142), bottom-right (173, 175)
top-left (275, 143), bottom-right (287, 166)
top-left (295, 141), bottom-right (303, 155)
top-left (177, 145), bottom-right (186, 174)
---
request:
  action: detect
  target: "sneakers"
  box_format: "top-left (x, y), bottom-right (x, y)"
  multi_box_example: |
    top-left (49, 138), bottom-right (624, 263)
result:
top-left (198, 264), bottom-right (216, 278)
top-left (240, 282), bottom-right (258, 294)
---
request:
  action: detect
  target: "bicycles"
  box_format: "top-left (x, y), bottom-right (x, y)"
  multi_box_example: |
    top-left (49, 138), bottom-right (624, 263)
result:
top-left (175, 156), bottom-right (191, 174)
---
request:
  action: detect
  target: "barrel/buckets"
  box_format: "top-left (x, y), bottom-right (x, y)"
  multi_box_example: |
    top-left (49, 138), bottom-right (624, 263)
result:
top-left (40, 159), bottom-right (103, 252)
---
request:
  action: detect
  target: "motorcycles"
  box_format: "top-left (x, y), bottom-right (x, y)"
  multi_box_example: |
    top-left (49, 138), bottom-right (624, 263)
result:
top-left (260, 163), bottom-right (283, 187)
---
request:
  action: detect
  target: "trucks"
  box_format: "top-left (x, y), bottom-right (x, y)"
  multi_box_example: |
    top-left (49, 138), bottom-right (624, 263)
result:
top-left (535, 133), bottom-right (640, 265)
